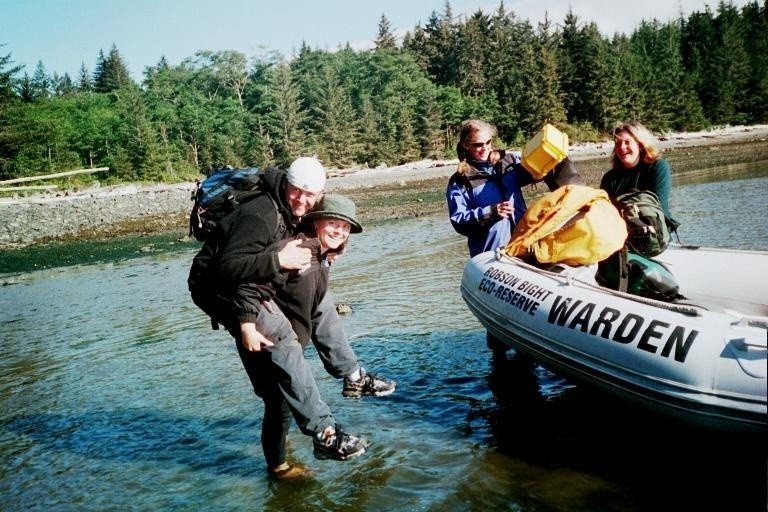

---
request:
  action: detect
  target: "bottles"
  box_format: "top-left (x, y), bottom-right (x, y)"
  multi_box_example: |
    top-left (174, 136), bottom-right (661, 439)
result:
top-left (286, 157), bottom-right (326, 195)
top-left (301, 194), bottom-right (364, 233)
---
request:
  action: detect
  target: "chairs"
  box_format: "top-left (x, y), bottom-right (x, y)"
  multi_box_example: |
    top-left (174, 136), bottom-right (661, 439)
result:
top-left (313, 423), bottom-right (368, 460)
top-left (343, 366), bottom-right (397, 398)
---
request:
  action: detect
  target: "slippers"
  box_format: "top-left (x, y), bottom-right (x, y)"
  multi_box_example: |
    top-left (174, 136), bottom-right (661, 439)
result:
top-left (613, 189), bottom-right (670, 257)
top-left (595, 252), bottom-right (679, 302)
top-left (193, 168), bottom-right (287, 242)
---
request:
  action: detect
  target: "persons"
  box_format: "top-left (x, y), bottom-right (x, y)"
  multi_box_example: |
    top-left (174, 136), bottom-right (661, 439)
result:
top-left (446, 119), bottom-right (532, 362)
top-left (235, 195), bottom-right (362, 482)
top-left (187, 156), bottom-right (397, 462)
top-left (600, 120), bottom-right (673, 242)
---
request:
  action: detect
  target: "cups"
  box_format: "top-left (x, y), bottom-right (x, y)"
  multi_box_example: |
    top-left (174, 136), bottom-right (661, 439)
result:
top-left (466, 139), bottom-right (492, 147)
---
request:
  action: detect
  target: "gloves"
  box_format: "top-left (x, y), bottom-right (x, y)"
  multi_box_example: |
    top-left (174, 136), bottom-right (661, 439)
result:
top-left (460, 242), bottom-right (768, 428)
